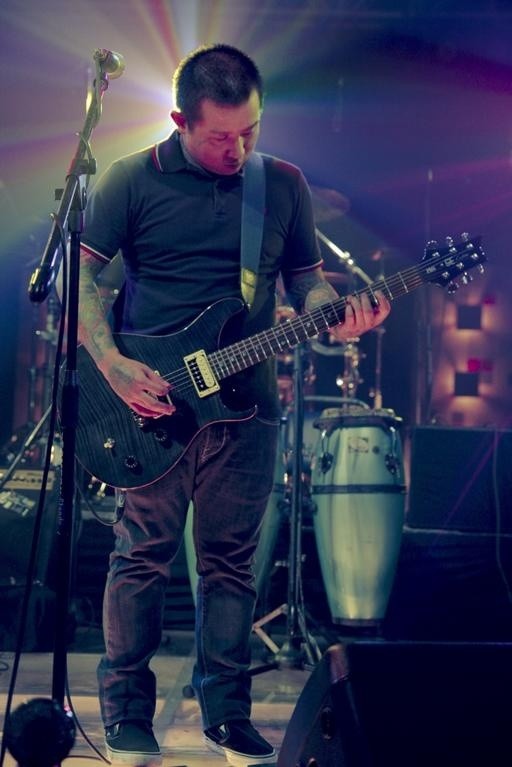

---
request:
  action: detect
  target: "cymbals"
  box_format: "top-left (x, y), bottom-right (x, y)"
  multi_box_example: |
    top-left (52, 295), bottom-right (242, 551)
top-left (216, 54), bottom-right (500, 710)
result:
top-left (309, 185), bottom-right (349, 226)
top-left (322, 271), bottom-right (347, 285)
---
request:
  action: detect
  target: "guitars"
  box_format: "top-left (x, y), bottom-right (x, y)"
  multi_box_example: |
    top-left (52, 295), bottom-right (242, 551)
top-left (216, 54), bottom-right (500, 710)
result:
top-left (55, 231), bottom-right (488, 491)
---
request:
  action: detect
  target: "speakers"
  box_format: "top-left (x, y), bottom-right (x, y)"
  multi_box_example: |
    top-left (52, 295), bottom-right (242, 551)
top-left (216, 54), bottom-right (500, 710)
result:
top-left (276, 640), bottom-right (511, 767)
top-left (407, 423), bottom-right (512, 531)
top-left (382, 527), bottom-right (510, 642)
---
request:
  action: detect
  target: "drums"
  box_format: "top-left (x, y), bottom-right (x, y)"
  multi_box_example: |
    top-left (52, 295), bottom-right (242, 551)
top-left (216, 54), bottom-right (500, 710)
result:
top-left (311, 406), bottom-right (407, 626)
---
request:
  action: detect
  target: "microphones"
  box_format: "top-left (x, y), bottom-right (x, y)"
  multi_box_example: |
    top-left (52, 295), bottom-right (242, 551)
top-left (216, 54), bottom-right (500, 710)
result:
top-left (93, 45), bottom-right (128, 80)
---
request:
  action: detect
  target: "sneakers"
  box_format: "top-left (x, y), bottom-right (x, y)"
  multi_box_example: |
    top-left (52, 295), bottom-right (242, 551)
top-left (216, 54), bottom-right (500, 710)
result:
top-left (104, 721), bottom-right (163, 767)
top-left (204, 717), bottom-right (277, 766)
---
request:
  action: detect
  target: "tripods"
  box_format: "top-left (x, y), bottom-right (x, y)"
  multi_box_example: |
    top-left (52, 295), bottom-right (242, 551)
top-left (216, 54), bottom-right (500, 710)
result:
top-left (182, 344), bottom-right (329, 702)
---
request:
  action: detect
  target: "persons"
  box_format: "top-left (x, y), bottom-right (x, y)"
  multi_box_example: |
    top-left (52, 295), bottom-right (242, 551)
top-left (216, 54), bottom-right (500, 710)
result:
top-left (53, 41), bottom-right (393, 767)
